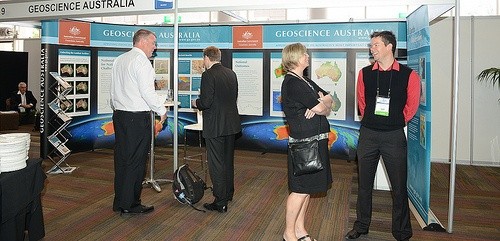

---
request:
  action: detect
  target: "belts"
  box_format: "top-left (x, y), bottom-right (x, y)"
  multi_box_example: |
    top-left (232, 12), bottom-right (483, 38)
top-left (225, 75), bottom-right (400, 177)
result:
top-left (113, 110), bottom-right (150, 115)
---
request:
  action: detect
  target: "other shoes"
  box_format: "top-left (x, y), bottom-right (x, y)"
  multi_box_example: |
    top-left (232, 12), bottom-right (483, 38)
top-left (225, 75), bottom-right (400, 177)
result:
top-left (344, 230), bottom-right (361, 239)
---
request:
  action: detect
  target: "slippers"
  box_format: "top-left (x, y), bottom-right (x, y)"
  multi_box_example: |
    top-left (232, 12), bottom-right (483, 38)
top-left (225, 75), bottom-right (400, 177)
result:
top-left (301, 235), bottom-right (318, 241)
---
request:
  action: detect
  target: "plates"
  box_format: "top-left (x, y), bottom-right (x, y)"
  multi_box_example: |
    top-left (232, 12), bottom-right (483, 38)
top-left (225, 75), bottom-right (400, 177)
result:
top-left (0, 133), bottom-right (32, 172)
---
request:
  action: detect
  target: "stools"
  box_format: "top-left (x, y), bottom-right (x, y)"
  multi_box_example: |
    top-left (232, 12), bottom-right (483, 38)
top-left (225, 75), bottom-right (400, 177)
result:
top-left (184, 109), bottom-right (209, 183)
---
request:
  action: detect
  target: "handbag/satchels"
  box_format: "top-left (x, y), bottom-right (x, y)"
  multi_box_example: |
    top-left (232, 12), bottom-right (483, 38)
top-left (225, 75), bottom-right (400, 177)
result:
top-left (289, 139), bottom-right (324, 177)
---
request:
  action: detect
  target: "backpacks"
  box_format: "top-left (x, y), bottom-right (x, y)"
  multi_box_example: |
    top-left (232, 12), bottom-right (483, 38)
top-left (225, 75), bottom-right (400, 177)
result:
top-left (172, 163), bottom-right (204, 206)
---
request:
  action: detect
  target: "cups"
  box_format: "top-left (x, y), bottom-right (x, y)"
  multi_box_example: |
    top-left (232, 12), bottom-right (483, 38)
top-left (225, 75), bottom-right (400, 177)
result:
top-left (168, 90), bottom-right (173, 102)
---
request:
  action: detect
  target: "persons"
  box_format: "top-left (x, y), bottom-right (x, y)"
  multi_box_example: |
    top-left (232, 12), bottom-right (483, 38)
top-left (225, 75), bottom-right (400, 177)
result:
top-left (192, 45), bottom-right (244, 214)
top-left (9, 82), bottom-right (38, 114)
top-left (279, 40), bottom-right (334, 241)
top-left (345, 29), bottom-right (422, 241)
top-left (108, 29), bottom-right (168, 215)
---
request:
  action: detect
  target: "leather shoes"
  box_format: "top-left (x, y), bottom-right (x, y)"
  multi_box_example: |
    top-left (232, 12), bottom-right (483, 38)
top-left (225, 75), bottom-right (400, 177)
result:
top-left (121, 204), bottom-right (155, 213)
top-left (203, 202), bottom-right (227, 213)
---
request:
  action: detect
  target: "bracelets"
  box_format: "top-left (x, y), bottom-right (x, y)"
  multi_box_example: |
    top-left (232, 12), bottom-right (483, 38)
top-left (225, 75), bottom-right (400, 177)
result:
top-left (317, 98), bottom-right (324, 102)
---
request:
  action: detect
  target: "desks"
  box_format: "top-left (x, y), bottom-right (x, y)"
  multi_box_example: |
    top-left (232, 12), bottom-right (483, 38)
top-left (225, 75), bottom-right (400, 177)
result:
top-left (142, 101), bottom-right (181, 192)
top-left (0, 111), bottom-right (19, 130)
top-left (0, 158), bottom-right (47, 241)
